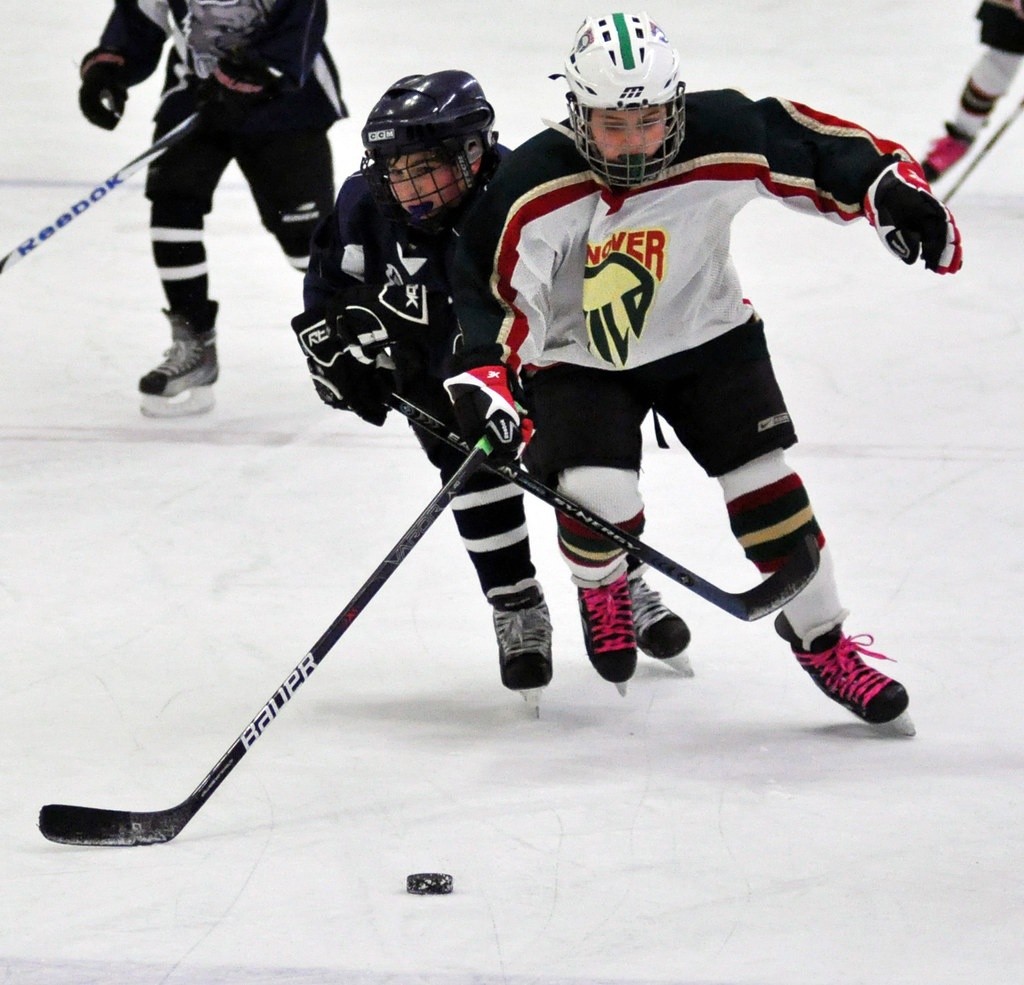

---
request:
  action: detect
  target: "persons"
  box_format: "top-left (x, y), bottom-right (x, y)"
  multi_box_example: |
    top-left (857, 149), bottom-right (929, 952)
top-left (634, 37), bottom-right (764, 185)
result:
top-left (78, 0), bottom-right (351, 421)
top-left (291, 69), bottom-right (696, 719)
top-left (922, 0), bottom-right (1024, 184)
top-left (441, 10), bottom-right (964, 738)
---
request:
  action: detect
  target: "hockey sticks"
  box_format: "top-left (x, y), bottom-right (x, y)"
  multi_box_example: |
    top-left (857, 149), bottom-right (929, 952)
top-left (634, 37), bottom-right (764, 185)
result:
top-left (37, 387), bottom-right (535, 847)
top-left (379, 386), bottom-right (823, 623)
top-left (941, 97), bottom-right (1022, 207)
top-left (1, 108), bottom-right (200, 279)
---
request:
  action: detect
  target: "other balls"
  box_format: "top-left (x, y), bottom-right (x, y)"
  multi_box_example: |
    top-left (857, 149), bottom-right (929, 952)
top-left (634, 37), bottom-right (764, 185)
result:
top-left (405, 871), bottom-right (455, 896)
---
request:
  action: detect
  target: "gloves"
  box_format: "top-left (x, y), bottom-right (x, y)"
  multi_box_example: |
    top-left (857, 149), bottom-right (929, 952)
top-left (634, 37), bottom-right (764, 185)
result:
top-left (862, 160), bottom-right (963, 274)
top-left (443, 366), bottom-right (534, 461)
top-left (199, 44), bottom-right (264, 129)
top-left (292, 307), bottom-right (401, 426)
top-left (78, 47), bottom-right (128, 131)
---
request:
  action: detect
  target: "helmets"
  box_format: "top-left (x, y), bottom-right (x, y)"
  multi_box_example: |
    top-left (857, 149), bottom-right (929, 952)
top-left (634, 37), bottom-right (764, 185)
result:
top-left (564, 11), bottom-right (686, 187)
top-left (361, 70), bottom-right (496, 249)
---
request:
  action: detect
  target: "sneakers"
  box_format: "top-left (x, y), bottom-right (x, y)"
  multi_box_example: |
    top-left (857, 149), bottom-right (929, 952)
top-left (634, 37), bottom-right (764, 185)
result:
top-left (774, 608), bottom-right (916, 737)
top-left (485, 577), bottom-right (553, 719)
top-left (921, 119), bottom-right (974, 182)
top-left (629, 577), bottom-right (695, 679)
top-left (570, 561), bottom-right (638, 698)
top-left (139, 299), bottom-right (218, 417)
